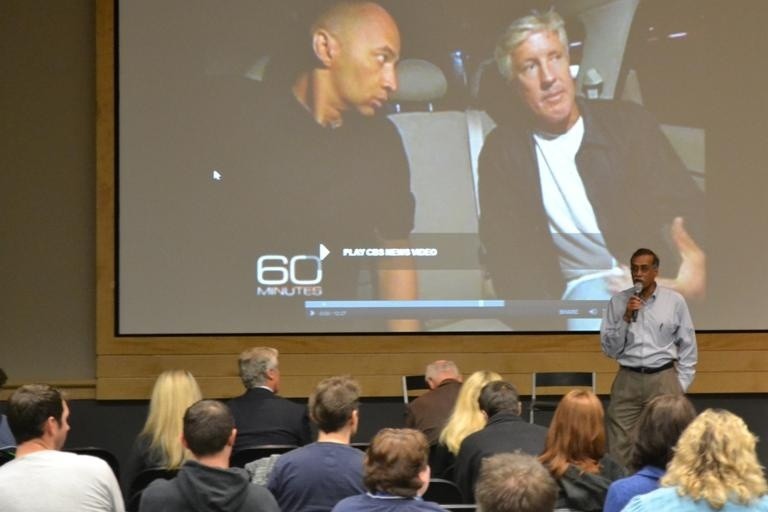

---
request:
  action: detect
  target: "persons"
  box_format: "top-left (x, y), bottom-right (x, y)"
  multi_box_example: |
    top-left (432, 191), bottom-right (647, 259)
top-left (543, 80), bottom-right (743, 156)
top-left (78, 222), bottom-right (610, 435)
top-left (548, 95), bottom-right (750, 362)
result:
top-left (204, 344), bottom-right (312, 468)
top-left (600, 249), bottom-right (698, 485)
top-left (407, 360), bottom-right (464, 449)
top-left (1, 376), bottom-right (767, 511)
top-left (476, 6), bottom-right (727, 332)
top-left (276, 0), bottom-right (425, 333)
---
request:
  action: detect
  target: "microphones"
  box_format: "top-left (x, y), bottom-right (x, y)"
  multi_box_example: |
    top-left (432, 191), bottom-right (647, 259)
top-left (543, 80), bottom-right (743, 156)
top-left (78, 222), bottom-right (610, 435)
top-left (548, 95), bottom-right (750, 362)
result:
top-left (631, 282), bottom-right (643, 322)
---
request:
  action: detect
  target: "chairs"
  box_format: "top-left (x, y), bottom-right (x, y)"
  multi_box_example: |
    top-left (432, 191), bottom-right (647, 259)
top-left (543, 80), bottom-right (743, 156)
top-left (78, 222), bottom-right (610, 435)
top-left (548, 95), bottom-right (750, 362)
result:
top-left (402, 375), bottom-right (432, 406)
top-left (426, 439), bottom-right (459, 484)
top-left (349, 402), bottom-right (406, 453)
top-left (127, 466), bottom-right (179, 512)
top-left (229, 445), bottom-right (300, 469)
top-left (62, 447), bottom-right (121, 480)
top-left (420, 477), bottom-right (468, 511)
top-left (0, 446), bottom-right (16, 467)
top-left (527, 371), bottom-right (597, 425)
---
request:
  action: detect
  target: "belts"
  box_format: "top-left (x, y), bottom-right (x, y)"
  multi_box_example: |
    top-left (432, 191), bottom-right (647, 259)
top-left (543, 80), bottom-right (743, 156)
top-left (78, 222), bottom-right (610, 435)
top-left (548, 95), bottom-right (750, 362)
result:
top-left (621, 361), bottom-right (673, 375)
